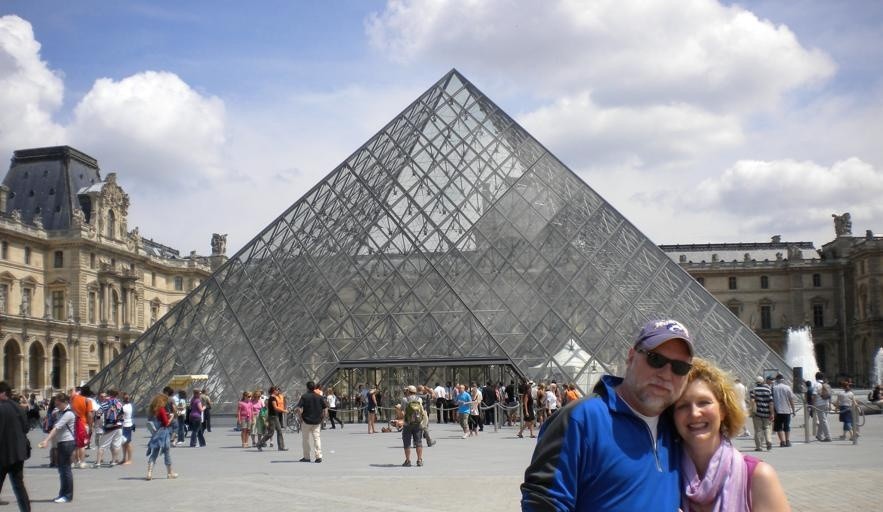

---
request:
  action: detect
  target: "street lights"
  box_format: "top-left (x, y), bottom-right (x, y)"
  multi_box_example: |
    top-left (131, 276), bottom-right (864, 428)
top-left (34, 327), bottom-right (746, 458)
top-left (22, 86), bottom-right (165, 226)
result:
top-left (49, 371), bottom-right (55, 400)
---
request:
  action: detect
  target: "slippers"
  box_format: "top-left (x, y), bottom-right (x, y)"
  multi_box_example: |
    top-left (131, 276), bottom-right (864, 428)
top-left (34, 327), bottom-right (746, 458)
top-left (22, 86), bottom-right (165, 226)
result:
top-left (516, 432), bottom-right (538, 439)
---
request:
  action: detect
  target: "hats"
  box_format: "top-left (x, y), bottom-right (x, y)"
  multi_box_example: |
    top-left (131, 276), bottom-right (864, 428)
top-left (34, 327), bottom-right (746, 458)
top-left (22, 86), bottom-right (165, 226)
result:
top-left (755, 376), bottom-right (764, 384)
top-left (404, 385), bottom-right (417, 393)
top-left (775, 374), bottom-right (784, 380)
top-left (634, 319), bottom-right (695, 358)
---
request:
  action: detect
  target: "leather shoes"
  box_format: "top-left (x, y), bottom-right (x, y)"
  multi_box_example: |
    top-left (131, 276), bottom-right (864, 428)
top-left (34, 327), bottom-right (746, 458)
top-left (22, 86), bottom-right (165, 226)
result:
top-left (300, 458), bottom-right (310, 462)
top-left (314, 458), bottom-right (322, 463)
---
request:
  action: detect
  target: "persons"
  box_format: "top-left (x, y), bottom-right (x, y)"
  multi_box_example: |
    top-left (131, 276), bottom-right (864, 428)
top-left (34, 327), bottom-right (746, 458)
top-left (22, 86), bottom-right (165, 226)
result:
top-left (65, 298), bottom-right (74, 322)
top-left (20, 292), bottom-right (30, 317)
top-left (671, 356), bottom-right (791, 512)
top-left (44, 294), bottom-right (54, 321)
top-left (71, 204), bottom-right (86, 225)
top-left (130, 225), bottom-right (141, 241)
top-left (0, 288), bottom-right (6, 316)
top-left (517, 319), bottom-right (695, 512)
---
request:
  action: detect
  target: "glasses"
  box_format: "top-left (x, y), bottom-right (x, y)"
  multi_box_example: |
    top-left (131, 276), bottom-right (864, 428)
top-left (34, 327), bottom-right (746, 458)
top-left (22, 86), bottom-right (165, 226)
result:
top-left (639, 347), bottom-right (693, 376)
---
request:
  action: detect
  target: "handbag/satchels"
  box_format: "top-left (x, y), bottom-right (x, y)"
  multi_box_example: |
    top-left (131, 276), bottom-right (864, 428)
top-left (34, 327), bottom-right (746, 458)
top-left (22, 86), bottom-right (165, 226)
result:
top-left (25, 435), bottom-right (32, 461)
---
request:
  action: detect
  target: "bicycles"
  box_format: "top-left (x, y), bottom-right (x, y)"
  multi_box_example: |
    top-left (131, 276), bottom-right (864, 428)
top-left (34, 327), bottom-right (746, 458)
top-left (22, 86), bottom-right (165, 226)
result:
top-left (286, 407), bottom-right (301, 434)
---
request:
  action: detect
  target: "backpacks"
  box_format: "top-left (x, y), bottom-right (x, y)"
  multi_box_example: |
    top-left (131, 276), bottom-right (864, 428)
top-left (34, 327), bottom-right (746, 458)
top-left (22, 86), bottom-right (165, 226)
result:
top-left (146, 414), bottom-right (161, 436)
top-left (62, 409), bottom-right (87, 447)
top-left (405, 395), bottom-right (424, 426)
top-left (816, 380), bottom-right (832, 400)
top-left (104, 399), bottom-right (119, 425)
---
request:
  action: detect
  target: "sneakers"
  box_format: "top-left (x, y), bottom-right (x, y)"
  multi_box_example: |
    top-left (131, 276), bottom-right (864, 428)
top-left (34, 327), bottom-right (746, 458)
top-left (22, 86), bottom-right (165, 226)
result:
top-left (754, 442), bottom-right (792, 451)
top-left (241, 441), bottom-right (289, 452)
top-left (401, 461), bottom-right (411, 466)
top-left (74, 461), bottom-right (132, 469)
top-left (816, 435), bottom-right (855, 443)
top-left (171, 441), bottom-right (184, 447)
top-left (427, 440), bottom-right (437, 447)
top-left (54, 496), bottom-right (68, 503)
top-left (167, 473), bottom-right (179, 479)
top-left (460, 431), bottom-right (480, 440)
top-left (417, 460), bottom-right (423, 466)
top-left (147, 474), bottom-right (152, 480)
